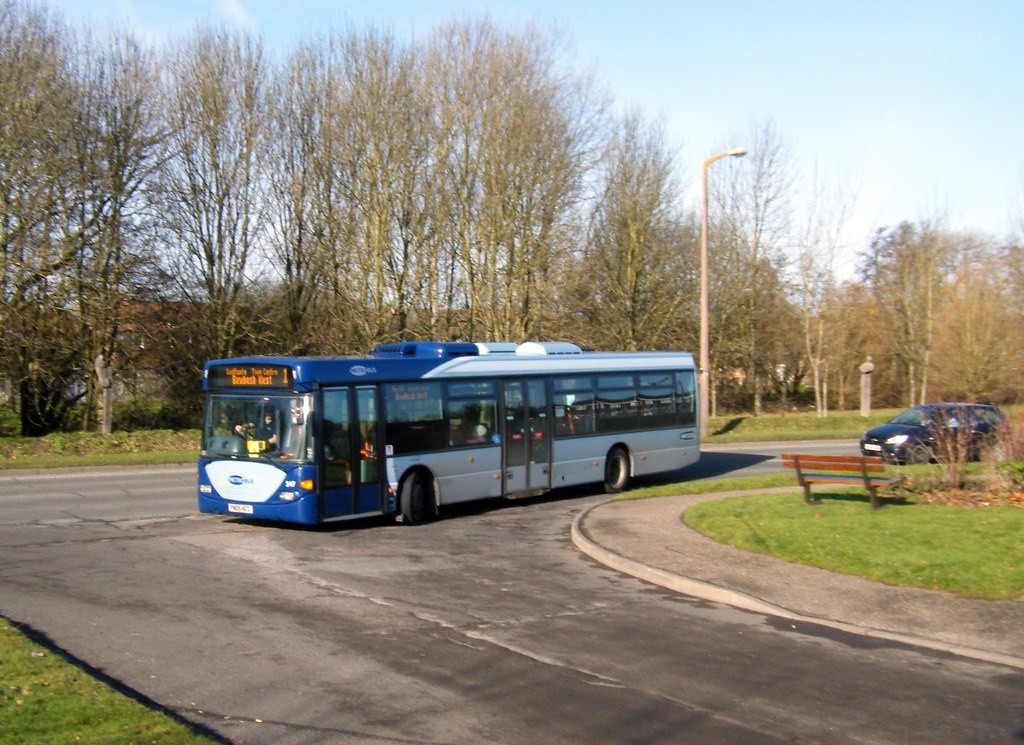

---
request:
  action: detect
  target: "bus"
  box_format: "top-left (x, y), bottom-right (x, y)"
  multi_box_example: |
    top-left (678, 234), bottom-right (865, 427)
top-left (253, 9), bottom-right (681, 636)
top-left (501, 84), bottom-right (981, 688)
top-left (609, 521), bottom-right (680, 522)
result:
top-left (198, 341), bottom-right (700, 525)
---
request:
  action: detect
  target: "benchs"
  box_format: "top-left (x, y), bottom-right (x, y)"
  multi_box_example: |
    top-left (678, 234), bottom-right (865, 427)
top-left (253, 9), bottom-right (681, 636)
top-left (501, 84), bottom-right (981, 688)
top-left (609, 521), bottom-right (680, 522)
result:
top-left (779, 451), bottom-right (897, 509)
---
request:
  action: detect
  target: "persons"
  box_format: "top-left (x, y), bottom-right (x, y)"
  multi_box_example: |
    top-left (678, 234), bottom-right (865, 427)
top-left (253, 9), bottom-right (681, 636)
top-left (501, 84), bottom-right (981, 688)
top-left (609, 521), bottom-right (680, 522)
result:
top-left (236, 407), bottom-right (278, 444)
top-left (562, 402), bottom-right (688, 434)
top-left (455, 387), bottom-right (490, 446)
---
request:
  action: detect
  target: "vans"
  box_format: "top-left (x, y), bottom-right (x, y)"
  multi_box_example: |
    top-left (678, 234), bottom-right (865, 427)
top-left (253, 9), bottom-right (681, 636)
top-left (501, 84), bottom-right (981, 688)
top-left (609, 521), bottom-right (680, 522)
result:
top-left (860, 401), bottom-right (1012, 464)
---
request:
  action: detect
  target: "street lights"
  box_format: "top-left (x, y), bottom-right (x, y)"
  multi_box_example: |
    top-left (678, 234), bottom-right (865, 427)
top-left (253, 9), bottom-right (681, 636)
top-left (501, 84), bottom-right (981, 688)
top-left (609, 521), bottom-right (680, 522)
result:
top-left (699, 149), bottom-right (748, 436)
